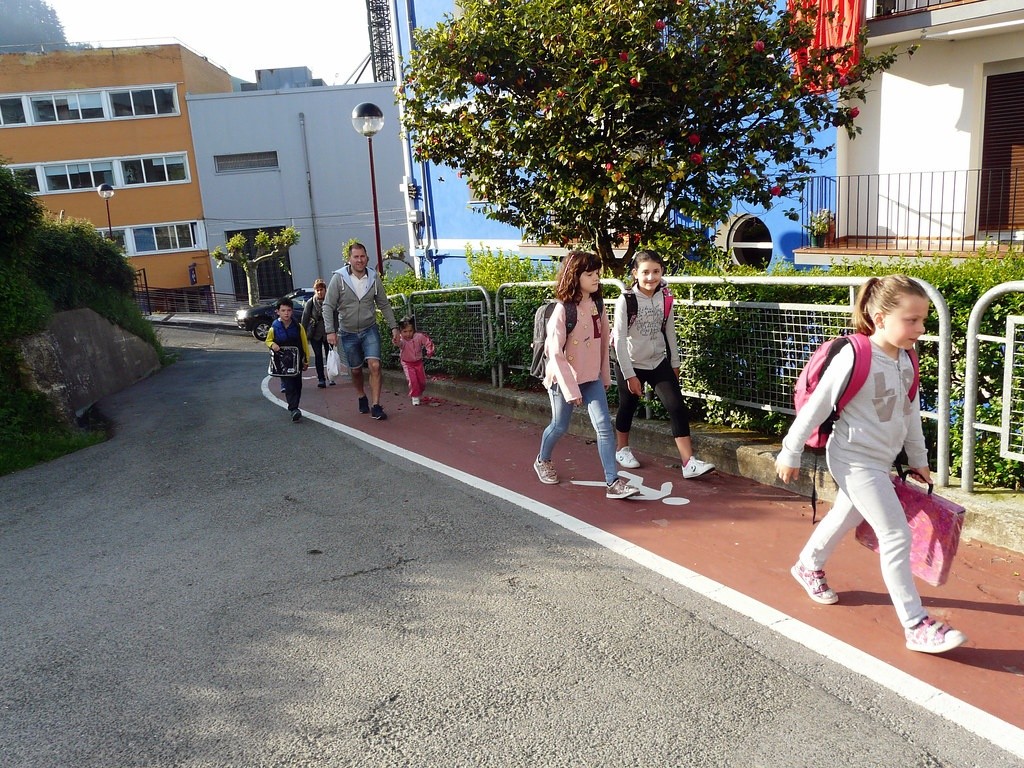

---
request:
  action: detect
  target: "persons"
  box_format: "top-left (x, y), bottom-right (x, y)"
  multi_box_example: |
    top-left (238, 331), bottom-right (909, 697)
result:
top-left (774, 274), bottom-right (967, 654)
top-left (321, 244), bottom-right (399, 419)
top-left (280, 377), bottom-right (286, 392)
top-left (265, 298), bottom-right (311, 421)
top-left (300, 279), bottom-right (339, 388)
top-left (391, 316), bottom-right (435, 406)
top-left (610, 250), bottom-right (715, 479)
top-left (533, 251), bottom-right (642, 498)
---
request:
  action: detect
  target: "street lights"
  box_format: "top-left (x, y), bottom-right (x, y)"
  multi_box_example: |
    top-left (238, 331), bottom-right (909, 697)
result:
top-left (349, 101), bottom-right (386, 282)
top-left (97, 184), bottom-right (115, 242)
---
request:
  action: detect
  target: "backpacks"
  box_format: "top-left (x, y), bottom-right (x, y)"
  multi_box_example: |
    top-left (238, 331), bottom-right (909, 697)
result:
top-left (529, 297), bottom-right (603, 380)
top-left (794, 333), bottom-right (919, 447)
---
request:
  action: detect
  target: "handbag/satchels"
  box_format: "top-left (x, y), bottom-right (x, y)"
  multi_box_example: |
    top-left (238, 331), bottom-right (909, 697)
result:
top-left (305, 297), bottom-right (316, 340)
top-left (268, 346), bottom-right (299, 376)
top-left (326, 343), bottom-right (341, 381)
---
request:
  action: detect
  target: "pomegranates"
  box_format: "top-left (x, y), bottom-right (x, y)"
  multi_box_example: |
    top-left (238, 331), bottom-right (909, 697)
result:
top-left (473, 18), bottom-right (860, 196)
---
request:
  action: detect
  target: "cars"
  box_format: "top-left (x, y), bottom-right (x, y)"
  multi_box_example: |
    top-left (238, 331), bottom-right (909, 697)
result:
top-left (234, 288), bottom-right (316, 341)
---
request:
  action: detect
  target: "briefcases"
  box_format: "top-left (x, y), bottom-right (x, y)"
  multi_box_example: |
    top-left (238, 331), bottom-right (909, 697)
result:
top-left (855, 470), bottom-right (964, 587)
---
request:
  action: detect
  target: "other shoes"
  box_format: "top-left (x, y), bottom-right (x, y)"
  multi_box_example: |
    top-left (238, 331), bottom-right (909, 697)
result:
top-left (281, 388), bottom-right (286, 393)
top-left (411, 397), bottom-right (420, 406)
top-left (317, 381), bottom-right (326, 388)
top-left (291, 409), bottom-right (301, 421)
top-left (328, 379), bottom-right (336, 385)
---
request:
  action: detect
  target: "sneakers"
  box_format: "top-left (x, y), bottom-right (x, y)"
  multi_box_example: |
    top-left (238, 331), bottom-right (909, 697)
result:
top-left (791, 559), bottom-right (838, 604)
top-left (534, 454), bottom-right (560, 484)
top-left (358, 395), bottom-right (370, 413)
top-left (681, 456), bottom-right (715, 478)
top-left (606, 478), bottom-right (640, 498)
top-left (615, 446), bottom-right (640, 468)
top-left (905, 618), bottom-right (967, 653)
top-left (371, 404), bottom-right (387, 420)
